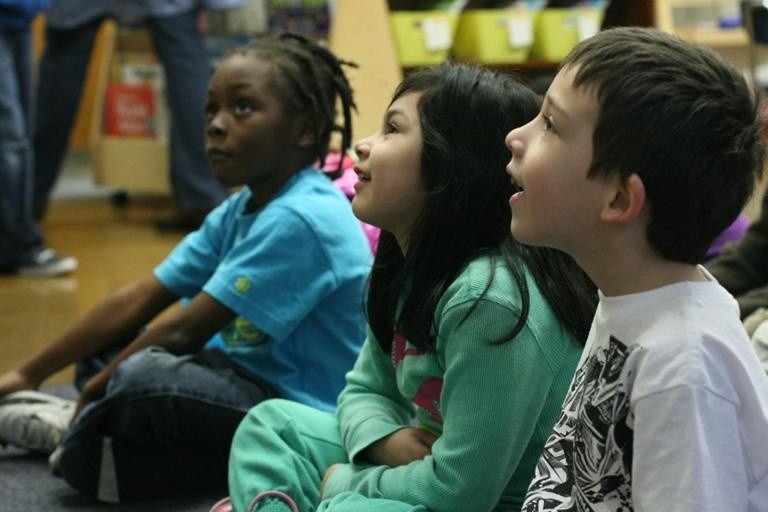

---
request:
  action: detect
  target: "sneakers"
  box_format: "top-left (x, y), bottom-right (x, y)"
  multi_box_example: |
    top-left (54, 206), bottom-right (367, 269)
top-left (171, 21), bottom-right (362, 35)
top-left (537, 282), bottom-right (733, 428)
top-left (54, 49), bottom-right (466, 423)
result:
top-left (14, 245), bottom-right (79, 278)
top-left (0, 388), bottom-right (78, 475)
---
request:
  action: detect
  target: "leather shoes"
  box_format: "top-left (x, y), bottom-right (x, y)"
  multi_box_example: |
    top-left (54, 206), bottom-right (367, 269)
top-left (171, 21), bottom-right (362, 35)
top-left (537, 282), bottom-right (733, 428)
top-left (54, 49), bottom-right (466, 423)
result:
top-left (154, 211), bottom-right (210, 232)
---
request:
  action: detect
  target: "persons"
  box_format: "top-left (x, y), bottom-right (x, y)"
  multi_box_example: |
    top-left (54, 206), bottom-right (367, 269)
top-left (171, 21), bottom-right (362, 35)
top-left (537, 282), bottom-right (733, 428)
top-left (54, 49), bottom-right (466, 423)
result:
top-left (1, 29), bottom-right (377, 510)
top-left (504, 26), bottom-right (767, 512)
top-left (702, 98), bottom-right (767, 373)
top-left (2, 1), bottom-right (76, 275)
top-left (212, 60), bottom-right (600, 510)
top-left (28, 0), bottom-right (229, 237)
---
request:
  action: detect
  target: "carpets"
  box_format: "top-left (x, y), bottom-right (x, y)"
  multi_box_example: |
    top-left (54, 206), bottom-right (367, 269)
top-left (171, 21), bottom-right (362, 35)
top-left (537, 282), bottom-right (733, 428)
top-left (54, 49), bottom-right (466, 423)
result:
top-left (1, 372), bottom-right (122, 511)
